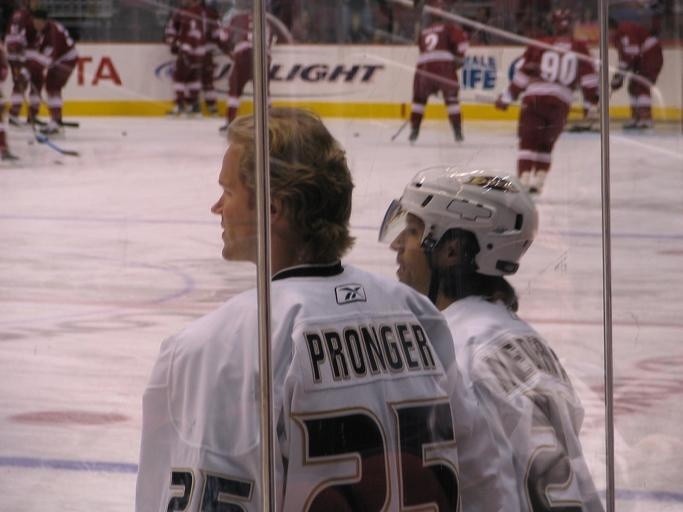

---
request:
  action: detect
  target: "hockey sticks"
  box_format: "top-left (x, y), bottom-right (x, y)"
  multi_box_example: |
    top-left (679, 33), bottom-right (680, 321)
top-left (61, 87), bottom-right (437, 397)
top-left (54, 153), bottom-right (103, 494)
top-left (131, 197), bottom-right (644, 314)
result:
top-left (31, 79), bottom-right (78, 127)
top-left (13, 67), bottom-right (78, 157)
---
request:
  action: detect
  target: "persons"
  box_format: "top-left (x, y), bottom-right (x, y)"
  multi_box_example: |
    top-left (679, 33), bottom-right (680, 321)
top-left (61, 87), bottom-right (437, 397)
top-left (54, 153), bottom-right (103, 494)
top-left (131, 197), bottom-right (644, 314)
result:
top-left (379, 166), bottom-right (602, 511)
top-left (605, 14), bottom-right (663, 129)
top-left (408, 1), bottom-right (470, 141)
top-left (348, 1), bottom-right (682, 46)
top-left (167, 2), bottom-right (311, 131)
top-left (0, 1), bottom-right (79, 160)
top-left (135, 107), bottom-right (474, 512)
top-left (495, 7), bottom-right (601, 193)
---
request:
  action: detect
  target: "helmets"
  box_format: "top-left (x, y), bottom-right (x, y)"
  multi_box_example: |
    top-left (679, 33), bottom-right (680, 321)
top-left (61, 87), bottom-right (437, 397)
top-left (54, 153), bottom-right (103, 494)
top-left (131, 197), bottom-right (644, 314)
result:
top-left (378, 165), bottom-right (539, 276)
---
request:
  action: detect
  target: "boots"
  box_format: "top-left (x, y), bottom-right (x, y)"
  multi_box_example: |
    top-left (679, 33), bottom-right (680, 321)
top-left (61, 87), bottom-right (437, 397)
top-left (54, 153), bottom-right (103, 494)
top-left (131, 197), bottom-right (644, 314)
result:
top-left (409, 131), bottom-right (418, 141)
top-left (623, 119), bottom-right (653, 130)
top-left (453, 133), bottom-right (463, 142)
